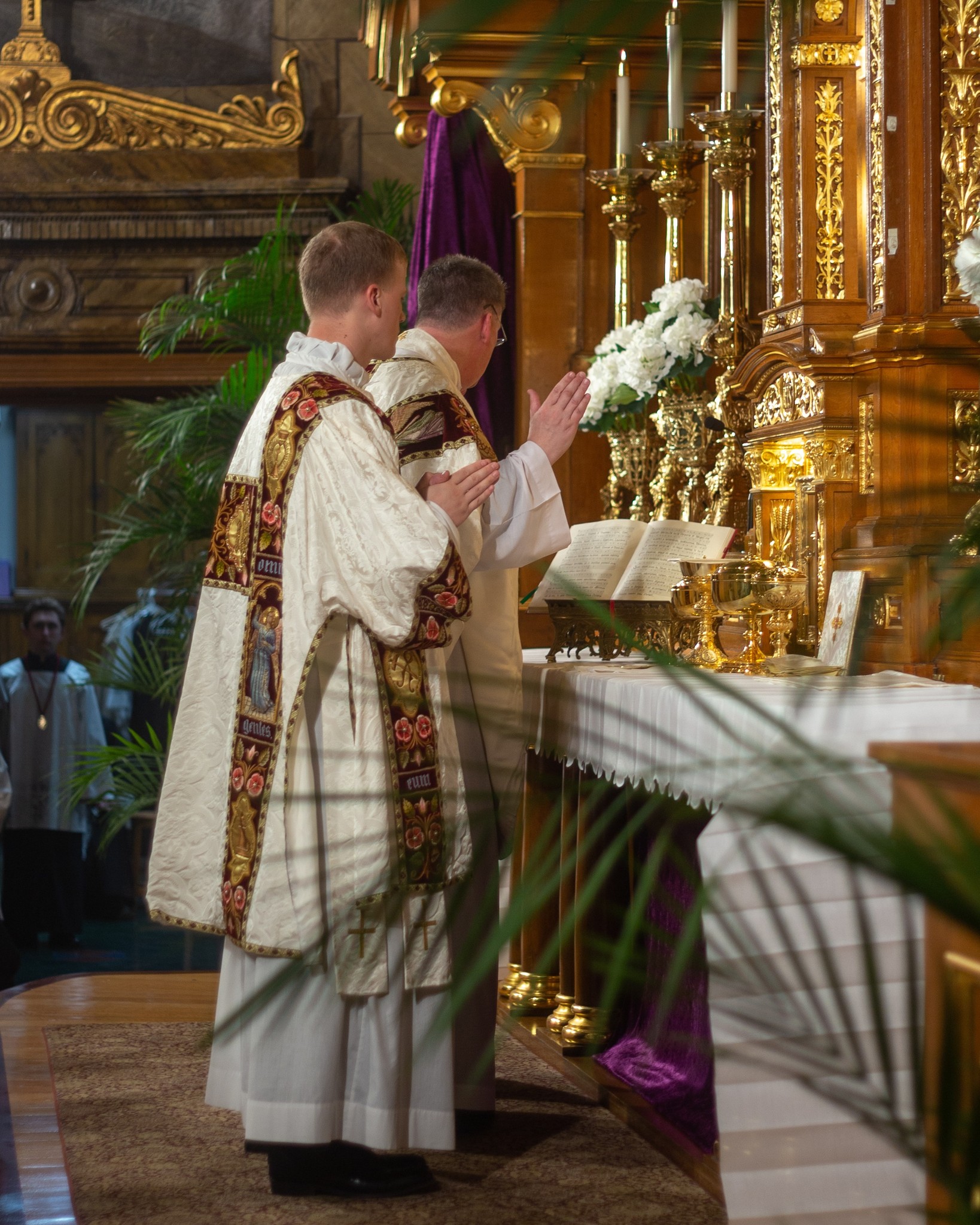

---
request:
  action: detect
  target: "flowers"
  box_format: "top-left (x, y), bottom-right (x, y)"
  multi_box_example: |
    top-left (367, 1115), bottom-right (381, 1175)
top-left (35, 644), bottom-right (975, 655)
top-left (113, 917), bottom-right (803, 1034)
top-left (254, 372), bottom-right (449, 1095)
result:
top-left (578, 277), bottom-right (714, 437)
top-left (953, 225), bottom-right (980, 311)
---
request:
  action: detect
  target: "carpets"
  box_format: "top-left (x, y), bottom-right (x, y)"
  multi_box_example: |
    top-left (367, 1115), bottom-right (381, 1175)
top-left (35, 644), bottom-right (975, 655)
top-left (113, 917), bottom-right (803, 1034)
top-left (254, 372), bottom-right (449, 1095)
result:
top-left (41, 1023), bottom-right (728, 1225)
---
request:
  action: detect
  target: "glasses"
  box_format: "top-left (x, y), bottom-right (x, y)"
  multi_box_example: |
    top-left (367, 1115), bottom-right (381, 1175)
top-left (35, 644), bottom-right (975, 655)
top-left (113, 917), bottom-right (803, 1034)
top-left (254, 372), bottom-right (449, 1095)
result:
top-left (483, 304), bottom-right (507, 347)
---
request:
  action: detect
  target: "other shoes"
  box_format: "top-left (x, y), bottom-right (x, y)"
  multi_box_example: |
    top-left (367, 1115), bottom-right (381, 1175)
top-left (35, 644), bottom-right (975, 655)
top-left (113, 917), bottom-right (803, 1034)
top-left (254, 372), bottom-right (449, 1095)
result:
top-left (269, 1139), bottom-right (435, 1197)
top-left (60, 921), bottom-right (85, 949)
top-left (86, 905), bottom-right (137, 923)
top-left (455, 1109), bottom-right (489, 1136)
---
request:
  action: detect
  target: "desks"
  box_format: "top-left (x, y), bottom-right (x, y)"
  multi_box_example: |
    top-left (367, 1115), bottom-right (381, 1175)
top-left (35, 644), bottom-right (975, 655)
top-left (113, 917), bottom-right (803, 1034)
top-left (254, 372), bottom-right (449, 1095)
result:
top-left (84, 793), bottom-right (194, 970)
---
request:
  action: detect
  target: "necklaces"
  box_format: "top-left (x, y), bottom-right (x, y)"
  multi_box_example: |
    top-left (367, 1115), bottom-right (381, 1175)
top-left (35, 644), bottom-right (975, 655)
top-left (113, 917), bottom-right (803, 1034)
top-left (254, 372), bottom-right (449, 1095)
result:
top-left (23, 654), bottom-right (60, 730)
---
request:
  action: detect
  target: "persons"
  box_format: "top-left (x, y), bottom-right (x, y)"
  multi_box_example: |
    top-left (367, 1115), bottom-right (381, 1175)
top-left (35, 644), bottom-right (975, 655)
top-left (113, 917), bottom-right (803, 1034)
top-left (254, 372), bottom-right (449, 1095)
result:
top-left (0, 596), bottom-right (115, 991)
top-left (147, 220), bottom-right (500, 1198)
top-left (366, 254), bottom-right (591, 1136)
